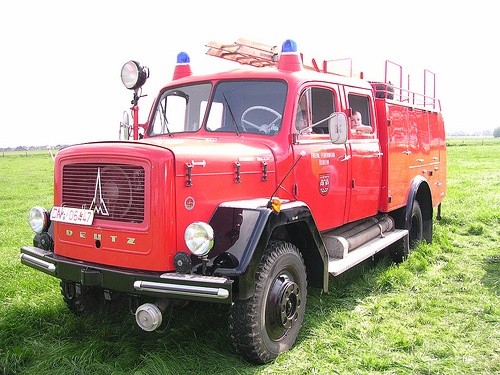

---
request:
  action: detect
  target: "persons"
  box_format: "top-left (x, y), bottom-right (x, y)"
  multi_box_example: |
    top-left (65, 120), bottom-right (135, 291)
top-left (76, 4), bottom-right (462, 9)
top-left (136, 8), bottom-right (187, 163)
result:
top-left (296, 110), bottom-right (315, 134)
top-left (351, 110), bottom-right (373, 133)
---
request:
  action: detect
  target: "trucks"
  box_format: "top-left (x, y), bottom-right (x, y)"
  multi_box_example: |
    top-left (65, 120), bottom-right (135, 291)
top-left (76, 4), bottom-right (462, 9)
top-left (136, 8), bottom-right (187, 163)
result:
top-left (17, 40), bottom-right (449, 366)
